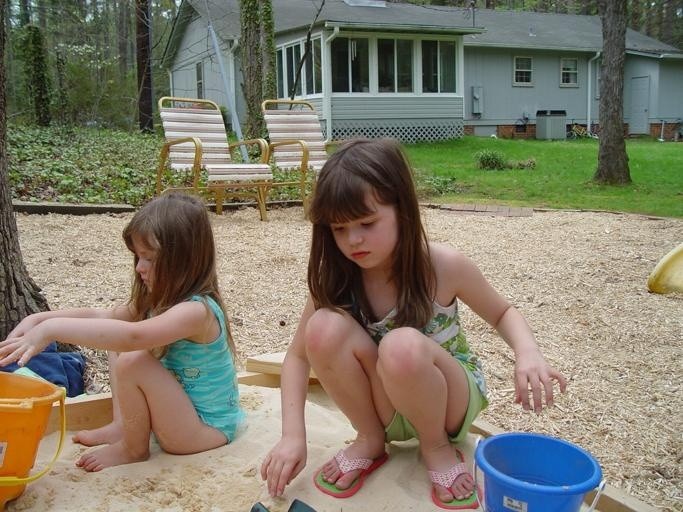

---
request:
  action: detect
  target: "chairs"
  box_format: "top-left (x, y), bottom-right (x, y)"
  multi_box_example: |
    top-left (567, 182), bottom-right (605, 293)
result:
top-left (154, 96), bottom-right (273, 222)
top-left (260, 97), bottom-right (343, 221)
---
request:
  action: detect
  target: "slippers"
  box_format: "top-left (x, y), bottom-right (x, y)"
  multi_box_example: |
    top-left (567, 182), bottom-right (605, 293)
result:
top-left (313, 448), bottom-right (390, 500)
top-left (427, 447), bottom-right (484, 510)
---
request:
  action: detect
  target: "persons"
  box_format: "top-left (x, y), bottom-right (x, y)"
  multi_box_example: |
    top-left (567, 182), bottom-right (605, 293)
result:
top-left (259, 136), bottom-right (567, 504)
top-left (1, 188), bottom-right (241, 473)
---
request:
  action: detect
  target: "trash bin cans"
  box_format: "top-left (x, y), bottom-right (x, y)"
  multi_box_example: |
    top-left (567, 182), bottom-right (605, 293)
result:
top-left (535, 110), bottom-right (567, 142)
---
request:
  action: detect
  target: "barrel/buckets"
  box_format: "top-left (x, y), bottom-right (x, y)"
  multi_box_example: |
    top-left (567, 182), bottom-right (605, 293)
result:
top-left (0, 372), bottom-right (66, 502)
top-left (647, 243), bottom-right (683, 295)
top-left (472, 432), bottom-right (606, 512)
